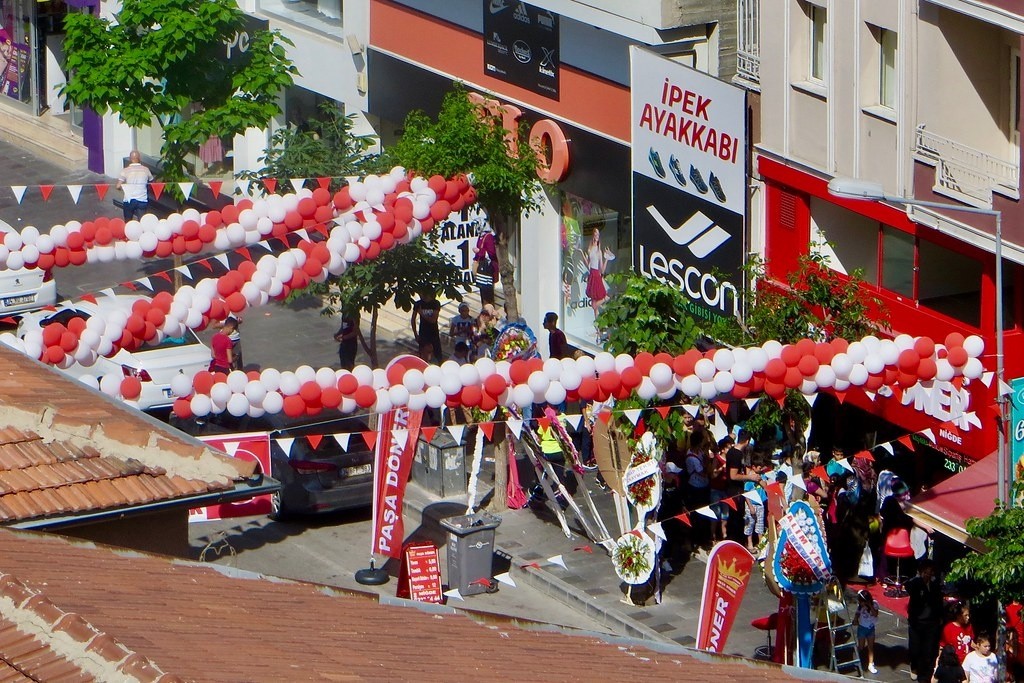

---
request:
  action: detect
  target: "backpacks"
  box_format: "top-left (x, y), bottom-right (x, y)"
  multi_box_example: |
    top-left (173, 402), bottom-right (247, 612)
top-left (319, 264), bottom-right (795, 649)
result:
top-left (682, 447), bottom-right (702, 483)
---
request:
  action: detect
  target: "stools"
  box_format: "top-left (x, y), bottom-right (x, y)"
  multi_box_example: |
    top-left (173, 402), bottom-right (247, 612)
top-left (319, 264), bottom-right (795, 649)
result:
top-left (883, 527), bottom-right (915, 598)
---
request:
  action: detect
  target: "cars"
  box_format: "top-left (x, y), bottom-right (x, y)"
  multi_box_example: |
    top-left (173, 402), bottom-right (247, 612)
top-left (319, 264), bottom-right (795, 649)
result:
top-left (0, 221), bottom-right (57, 315)
top-left (19, 294), bottom-right (216, 413)
top-left (170, 411), bottom-right (374, 514)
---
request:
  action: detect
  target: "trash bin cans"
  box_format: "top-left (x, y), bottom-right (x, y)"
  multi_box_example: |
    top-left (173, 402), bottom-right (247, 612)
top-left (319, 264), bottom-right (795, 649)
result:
top-left (439, 510), bottom-right (499, 596)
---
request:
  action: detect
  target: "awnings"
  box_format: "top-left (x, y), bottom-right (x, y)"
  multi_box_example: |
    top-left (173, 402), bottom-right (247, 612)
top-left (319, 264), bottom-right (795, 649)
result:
top-left (902, 442), bottom-right (1011, 555)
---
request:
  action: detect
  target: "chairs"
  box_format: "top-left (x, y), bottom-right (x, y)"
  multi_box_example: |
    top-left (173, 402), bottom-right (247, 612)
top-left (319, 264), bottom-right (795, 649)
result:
top-left (750, 612), bottom-right (779, 660)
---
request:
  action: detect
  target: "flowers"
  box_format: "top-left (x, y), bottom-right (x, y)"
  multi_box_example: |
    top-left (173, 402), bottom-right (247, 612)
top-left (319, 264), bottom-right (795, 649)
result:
top-left (627, 438), bottom-right (657, 511)
top-left (611, 534), bottom-right (653, 583)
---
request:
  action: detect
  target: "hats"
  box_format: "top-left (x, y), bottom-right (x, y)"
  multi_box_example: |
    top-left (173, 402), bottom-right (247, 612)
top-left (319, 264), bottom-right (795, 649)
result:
top-left (666, 462), bottom-right (683, 473)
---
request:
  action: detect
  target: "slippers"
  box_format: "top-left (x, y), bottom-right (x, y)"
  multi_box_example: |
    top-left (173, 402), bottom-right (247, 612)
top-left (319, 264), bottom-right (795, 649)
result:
top-left (747, 547), bottom-right (756, 553)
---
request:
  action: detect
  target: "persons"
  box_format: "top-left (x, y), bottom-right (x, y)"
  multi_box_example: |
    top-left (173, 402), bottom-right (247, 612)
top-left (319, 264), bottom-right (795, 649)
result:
top-left (662, 412), bottom-right (1024, 683)
top-left (411, 289), bottom-right (526, 365)
top-left (471, 216), bottom-right (499, 306)
top-left (543, 312), bottom-right (568, 359)
top-left (116, 151), bottom-right (154, 222)
top-left (578, 228), bottom-right (609, 320)
top-left (209, 311), bottom-right (243, 376)
top-left (520, 398), bottom-right (615, 509)
top-left (334, 314), bottom-right (360, 372)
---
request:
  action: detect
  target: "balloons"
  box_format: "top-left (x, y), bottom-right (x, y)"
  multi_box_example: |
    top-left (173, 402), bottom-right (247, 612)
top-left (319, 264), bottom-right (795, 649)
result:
top-left (0, 168), bottom-right (479, 369)
top-left (80, 333), bottom-right (984, 417)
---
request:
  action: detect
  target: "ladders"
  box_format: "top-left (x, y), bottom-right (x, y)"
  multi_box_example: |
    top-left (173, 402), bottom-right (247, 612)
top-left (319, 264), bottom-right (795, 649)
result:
top-left (808, 575), bottom-right (865, 678)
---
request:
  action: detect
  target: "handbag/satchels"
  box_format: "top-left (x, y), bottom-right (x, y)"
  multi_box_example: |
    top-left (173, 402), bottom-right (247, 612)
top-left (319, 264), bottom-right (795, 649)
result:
top-left (857, 541), bottom-right (873, 577)
top-left (604, 246), bottom-right (615, 260)
top-left (475, 250), bottom-right (495, 291)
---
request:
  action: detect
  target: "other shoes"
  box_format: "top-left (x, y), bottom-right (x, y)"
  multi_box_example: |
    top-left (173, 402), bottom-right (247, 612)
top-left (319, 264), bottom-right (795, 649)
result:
top-left (662, 561), bottom-right (672, 571)
top-left (582, 460), bottom-right (598, 469)
top-left (596, 479), bottom-right (606, 490)
top-left (867, 664), bottom-right (878, 674)
top-left (909, 662), bottom-right (918, 680)
